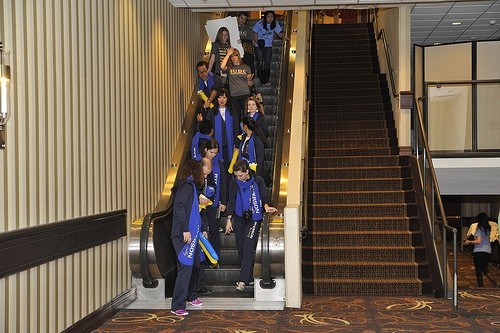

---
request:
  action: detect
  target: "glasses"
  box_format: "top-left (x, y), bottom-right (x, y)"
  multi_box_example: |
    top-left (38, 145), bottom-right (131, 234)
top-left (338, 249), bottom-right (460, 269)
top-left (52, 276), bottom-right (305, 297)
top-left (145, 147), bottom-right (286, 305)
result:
top-left (198, 69), bottom-right (207, 73)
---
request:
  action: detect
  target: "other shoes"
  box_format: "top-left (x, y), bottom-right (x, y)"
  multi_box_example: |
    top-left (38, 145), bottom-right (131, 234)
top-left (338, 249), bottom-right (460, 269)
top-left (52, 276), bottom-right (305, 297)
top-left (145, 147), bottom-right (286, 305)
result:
top-left (236, 282), bottom-right (245, 291)
top-left (197, 289), bottom-right (212, 295)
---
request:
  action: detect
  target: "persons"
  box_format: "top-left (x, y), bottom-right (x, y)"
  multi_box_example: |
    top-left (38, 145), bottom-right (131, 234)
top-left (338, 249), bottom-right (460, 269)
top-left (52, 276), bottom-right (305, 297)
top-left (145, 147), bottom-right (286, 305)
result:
top-left (207, 27), bottom-right (232, 77)
top-left (170, 159), bottom-right (207, 316)
top-left (252, 10), bottom-right (285, 87)
top-left (190, 48), bottom-right (277, 295)
top-left (464, 212), bottom-right (500, 288)
top-left (236, 12), bottom-right (254, 74)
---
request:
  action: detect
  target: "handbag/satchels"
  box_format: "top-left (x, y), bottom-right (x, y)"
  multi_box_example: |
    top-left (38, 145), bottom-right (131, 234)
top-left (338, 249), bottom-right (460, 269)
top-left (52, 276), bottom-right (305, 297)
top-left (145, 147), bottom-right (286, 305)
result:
top-left (250, 85), bottom-right (264, 115)
top-left (261, 171), bottom-right (272, 186)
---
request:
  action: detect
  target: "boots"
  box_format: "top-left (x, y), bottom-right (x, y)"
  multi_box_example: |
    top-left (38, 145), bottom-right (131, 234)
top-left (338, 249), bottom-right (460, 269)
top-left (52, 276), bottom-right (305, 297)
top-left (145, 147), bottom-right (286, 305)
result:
top-left (485, 273), bottom-right (497, 288)
top-left (475, 275), bottom-right (484, 288)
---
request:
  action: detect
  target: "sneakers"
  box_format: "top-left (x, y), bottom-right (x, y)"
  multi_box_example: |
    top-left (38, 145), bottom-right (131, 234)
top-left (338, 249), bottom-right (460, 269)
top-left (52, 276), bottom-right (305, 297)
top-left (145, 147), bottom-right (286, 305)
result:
top-left (190, 299), bottom-right (203, 306)
top-left (171, 309), bottom-right (189, 315)
